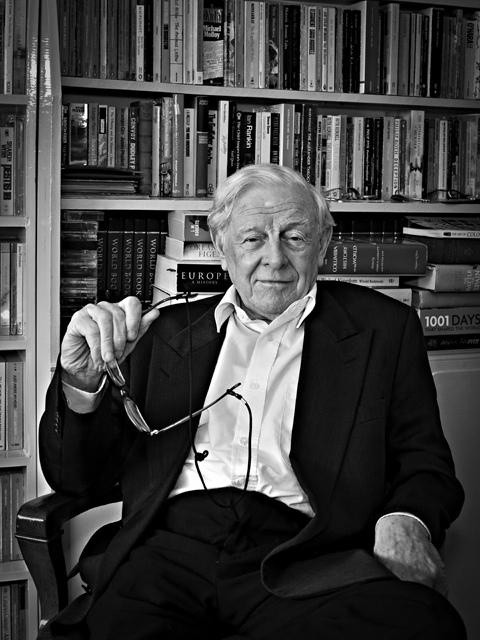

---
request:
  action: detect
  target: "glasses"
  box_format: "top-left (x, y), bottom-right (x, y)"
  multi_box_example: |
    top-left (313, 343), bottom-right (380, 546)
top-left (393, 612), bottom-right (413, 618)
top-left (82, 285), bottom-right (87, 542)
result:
top-left (106, 293), bottom-right (241, 435)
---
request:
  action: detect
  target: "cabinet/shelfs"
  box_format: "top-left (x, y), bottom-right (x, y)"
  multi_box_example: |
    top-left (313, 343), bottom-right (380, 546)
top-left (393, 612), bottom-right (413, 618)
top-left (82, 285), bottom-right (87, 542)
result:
top-left (0, 0), bottom-right (37, 640)
top-left (37, 0), bottom-right (480, 364)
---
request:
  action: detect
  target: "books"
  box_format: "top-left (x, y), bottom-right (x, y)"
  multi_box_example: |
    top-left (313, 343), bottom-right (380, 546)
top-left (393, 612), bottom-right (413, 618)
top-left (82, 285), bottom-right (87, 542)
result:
top-left (60, 208), bottom-right (233, 332)
top-left (60, 92), bottom-right (480, 200)
top-left (315, 211), bottom-right (479, 339)
top-left (61, 0), bottom-right (480, 100)
top-left (0, 0), bottom-right (30, 640)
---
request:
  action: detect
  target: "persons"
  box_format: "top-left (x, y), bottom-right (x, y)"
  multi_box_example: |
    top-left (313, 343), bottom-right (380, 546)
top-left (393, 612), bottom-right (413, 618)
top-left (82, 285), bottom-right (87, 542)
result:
top-left (39, 164), bottom-right (466, 638)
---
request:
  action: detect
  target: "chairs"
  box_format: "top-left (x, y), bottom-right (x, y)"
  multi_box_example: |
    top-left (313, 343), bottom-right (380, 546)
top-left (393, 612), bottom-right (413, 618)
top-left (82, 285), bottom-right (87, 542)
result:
top-left (13, 482), bottom-right (123, 629)
